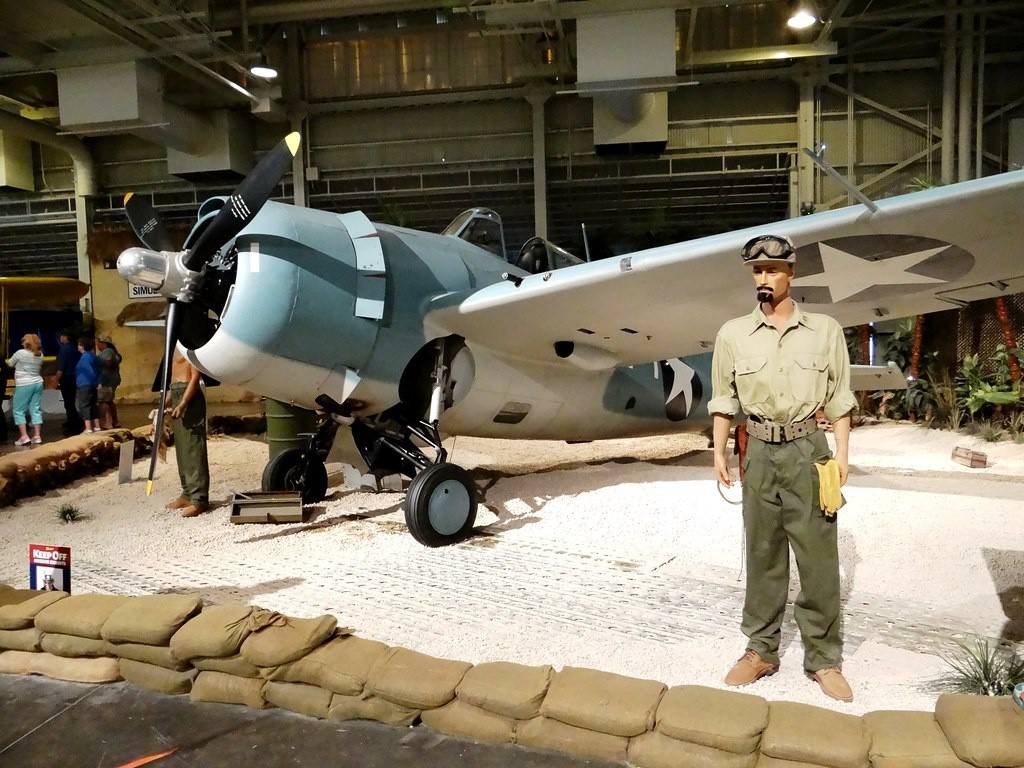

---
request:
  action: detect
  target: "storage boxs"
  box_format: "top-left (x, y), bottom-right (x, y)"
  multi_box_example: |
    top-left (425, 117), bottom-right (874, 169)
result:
top-left (950, 444), bottom-right (989, 469)
top-left (228, 488), bottom-right (305, 525)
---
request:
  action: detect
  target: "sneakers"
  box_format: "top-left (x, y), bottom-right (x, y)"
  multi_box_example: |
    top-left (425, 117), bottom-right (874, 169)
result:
top-left (725, 650), bottom-right (779, 685)
top-left (181, 504), bottom-right (209, 516)
top-left (165, 497), bottom-right (191, 509)
top-left (803, 667), bottom-right (854, 703)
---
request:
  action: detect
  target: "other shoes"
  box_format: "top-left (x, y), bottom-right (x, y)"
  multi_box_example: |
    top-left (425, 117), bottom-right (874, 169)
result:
top-left (15, 438), bottom-right (31, 446)
top-left (101, 427), bottom-right (109, 430)
top-left (113, 426), bottom-right (121, 428)
top-left (31, 436), bottom-right (42, 444)
top-left (81, 430), bottom-right (93, 434)
top-left (94, 428), bottom-right (101, 432)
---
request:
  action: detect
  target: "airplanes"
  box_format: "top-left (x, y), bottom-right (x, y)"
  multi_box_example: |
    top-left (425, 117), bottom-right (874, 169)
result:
top-left (108, 132), bottom-right (1024, 549)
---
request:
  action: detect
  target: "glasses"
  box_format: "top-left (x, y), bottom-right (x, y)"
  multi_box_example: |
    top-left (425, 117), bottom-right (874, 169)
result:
top-left (741, 235), bottom-right (795, 260)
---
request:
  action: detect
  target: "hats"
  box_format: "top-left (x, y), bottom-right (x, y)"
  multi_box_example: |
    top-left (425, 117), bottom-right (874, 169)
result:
top-left (742, 235), bottom-right (797, 266)
top-left (99, 335), bottom-right (112, 343)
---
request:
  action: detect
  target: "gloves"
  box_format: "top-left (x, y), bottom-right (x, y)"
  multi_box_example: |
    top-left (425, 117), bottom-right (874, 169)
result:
top-left (815, 460), bottom-right (842, 517)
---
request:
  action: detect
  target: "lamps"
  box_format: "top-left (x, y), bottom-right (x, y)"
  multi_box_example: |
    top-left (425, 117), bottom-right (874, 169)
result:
top-left (244, 44), bottom-right (279, 79)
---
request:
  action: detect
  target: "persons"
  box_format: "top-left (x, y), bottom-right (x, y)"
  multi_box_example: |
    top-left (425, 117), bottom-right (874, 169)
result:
top-left (164, 347), bottom-right (210, 518)
top-left (7, 333), bottom-right (44, 446)
top-left (51, 327), bottom-right (83, 437)
top-left (707, 234), bottom-right (860, 704)
top-left (73, 336), bottom-right (101, 436)
top-left (40, 575), bottom-right (61, 591)
top-left (96, 334), bottom-right (123, 429)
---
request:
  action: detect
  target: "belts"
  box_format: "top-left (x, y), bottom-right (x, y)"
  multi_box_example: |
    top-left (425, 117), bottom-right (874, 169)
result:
top-left (170, 384), bottom-right (188, 390)
top-left (746, 417), bottom-right (816, 442)
